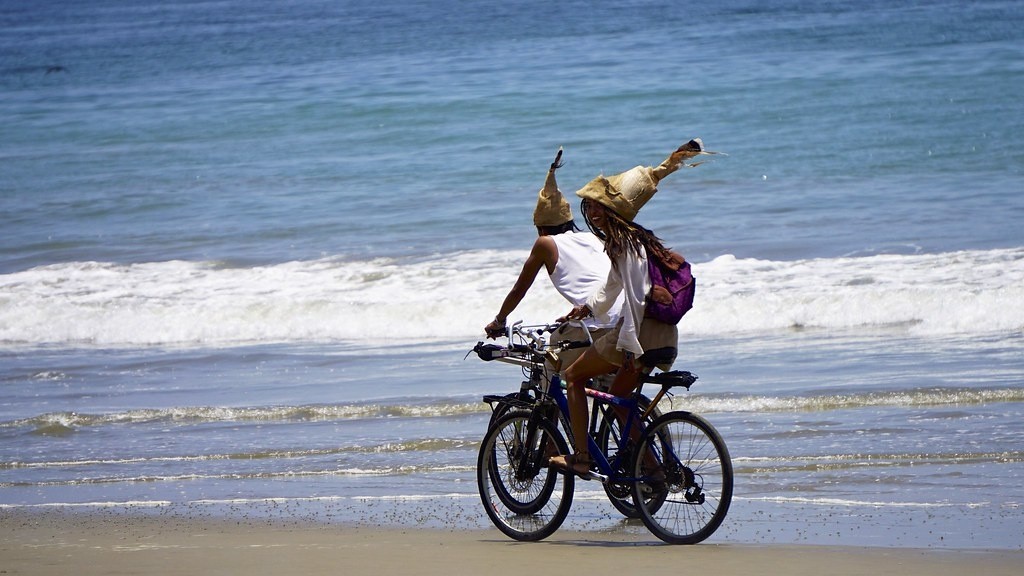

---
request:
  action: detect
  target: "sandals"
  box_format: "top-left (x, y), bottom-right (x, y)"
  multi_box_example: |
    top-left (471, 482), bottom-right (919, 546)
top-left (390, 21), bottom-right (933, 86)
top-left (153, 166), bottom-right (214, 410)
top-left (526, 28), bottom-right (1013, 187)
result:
top-left (549, 454), bottom-right (592, 481)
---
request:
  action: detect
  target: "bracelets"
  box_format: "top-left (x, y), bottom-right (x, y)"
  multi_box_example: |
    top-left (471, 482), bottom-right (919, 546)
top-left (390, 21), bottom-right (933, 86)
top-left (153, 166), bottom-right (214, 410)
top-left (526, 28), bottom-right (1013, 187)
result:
top-left (494, 315), bottom-right (508, 325)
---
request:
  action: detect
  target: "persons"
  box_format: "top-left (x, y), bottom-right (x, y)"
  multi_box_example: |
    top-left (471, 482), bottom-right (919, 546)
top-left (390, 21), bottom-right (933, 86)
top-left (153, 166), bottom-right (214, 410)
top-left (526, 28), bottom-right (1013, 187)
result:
top-left (545, 185), bottom-right (678, 489)
top-left (485, 201), bottom-right (627, 459)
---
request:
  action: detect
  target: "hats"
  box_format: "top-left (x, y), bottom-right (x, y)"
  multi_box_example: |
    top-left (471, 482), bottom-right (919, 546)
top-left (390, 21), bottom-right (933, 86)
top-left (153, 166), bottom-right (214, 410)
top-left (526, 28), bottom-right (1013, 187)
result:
top-left (533, 146), bottom-right (574, 227)
top-left (575, 138), bottom-right (727, 222)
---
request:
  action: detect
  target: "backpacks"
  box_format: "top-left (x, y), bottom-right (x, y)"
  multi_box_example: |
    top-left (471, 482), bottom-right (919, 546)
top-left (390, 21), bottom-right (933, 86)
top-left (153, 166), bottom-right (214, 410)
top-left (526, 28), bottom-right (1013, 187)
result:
top-left (643, 243), bottom-right (696, 326)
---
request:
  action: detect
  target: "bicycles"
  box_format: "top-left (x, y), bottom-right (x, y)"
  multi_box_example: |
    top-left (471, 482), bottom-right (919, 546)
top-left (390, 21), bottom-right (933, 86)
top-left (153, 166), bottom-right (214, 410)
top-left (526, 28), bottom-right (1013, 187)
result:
top-left (463, 317), bottom-right (734, 547)
